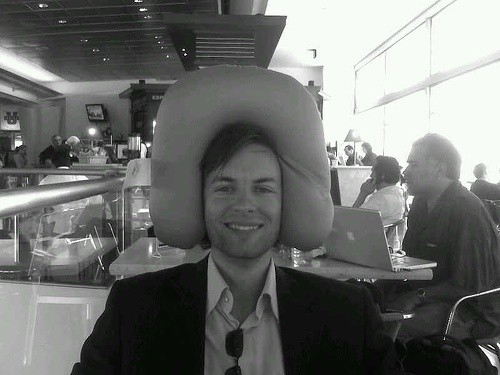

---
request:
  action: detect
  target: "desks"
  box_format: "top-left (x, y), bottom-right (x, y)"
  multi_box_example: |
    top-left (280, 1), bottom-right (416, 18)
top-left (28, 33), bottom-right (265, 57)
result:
top-left (110, 236), bottom-right (433, 281)
top-left (332, 166), bottom-right (374, 206)
top-left (0, 239), bottom-right (116, 275)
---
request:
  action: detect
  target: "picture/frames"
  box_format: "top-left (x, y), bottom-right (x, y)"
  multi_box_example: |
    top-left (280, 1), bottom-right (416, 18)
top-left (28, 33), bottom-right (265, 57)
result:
top-left (85, 104), bottom-right (106, 122)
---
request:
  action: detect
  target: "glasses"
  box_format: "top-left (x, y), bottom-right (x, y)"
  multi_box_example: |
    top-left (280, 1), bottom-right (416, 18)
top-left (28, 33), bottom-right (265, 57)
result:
top-left (225, 324), bottom-right (243, 375)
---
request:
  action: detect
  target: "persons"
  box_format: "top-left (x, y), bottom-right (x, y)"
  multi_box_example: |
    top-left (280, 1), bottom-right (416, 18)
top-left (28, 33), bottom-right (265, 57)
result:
top-left (357, 141), bottom-right (378, 166)
top-left (362, 133), bottom-right (500, 374)
top-left (39, 135), bottom-right (62, 165)
top-left (351, 155), bottom-right (406, 252)
top-left (88, 140), bottom-right (111, 164)
top-left (470, 162), bottom-right (500, 200)
top-left (52, 136), bottom-right (80, 168)
top-left (70, 121), bottom-right (407, 375)
top-left (344, 144), bottom-right (364, 166)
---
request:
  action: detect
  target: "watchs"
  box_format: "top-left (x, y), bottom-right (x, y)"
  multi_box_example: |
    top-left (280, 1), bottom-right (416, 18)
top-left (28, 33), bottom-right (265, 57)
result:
top-left (416, 285), bottom-right (429, 302)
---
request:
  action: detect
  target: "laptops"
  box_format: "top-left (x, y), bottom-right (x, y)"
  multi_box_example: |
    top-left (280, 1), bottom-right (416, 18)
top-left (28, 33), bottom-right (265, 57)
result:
top-left (327, 206), bottom-right (437, 272)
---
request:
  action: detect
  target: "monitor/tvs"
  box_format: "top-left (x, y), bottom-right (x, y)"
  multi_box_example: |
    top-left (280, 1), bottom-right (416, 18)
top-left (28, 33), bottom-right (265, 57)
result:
top-left (86, 104), bottom-right (104, 121)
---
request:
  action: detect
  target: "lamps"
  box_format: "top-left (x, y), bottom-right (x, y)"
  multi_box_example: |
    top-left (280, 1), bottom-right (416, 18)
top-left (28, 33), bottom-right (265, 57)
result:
top-left (344, 129), bottom-right (362, 164)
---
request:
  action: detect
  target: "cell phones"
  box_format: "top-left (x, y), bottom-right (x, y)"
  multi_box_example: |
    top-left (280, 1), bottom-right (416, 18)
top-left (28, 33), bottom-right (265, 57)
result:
top-left (376, 174), bottom-right (384, 183)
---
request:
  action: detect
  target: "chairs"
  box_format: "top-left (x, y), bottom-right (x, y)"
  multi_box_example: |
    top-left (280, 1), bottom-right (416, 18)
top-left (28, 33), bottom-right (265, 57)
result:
top-left (446, 287), bottom-right (500, 361)
top-left (78, 218), bottom-right (117, 280)
